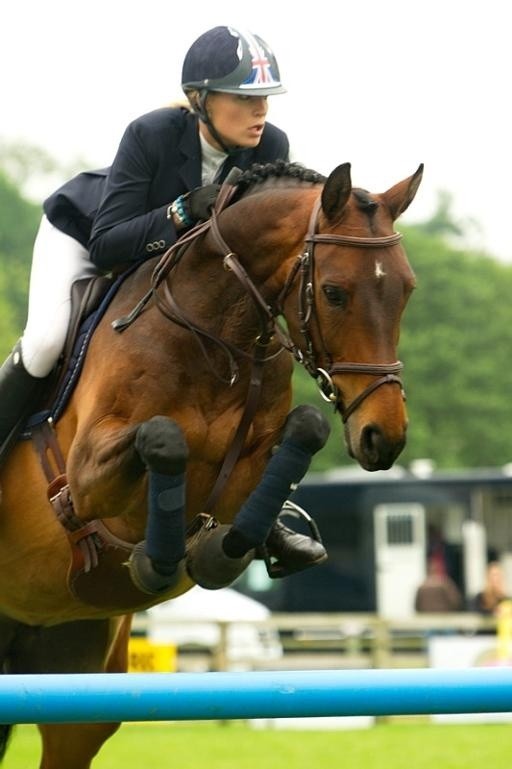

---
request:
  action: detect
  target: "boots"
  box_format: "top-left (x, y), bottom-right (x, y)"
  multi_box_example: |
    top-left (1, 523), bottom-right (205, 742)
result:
top-left (254, 517), bottom-right (328, 567)
top-left (0, 337), bottom-right (61, 444)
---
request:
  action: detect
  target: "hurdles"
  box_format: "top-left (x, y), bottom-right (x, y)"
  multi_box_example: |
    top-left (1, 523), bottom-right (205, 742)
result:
top-left (0, 667), bottom-right (512, 724)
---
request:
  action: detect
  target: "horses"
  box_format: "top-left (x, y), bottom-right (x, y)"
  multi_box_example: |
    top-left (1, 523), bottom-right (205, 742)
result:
top-left (0, 157), bottom-right (428, 768)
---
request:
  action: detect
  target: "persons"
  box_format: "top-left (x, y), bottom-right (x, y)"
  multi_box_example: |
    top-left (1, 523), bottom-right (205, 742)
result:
top-left (472, 562), bottom-right (507, 636)
top-left (0, 25), bottom-right (290, 441)
top-left (412, 559), bottom-right (463, 657)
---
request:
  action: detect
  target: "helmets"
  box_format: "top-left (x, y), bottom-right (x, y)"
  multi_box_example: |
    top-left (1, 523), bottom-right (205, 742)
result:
top-left (182, 25), bottom-right (287, 96)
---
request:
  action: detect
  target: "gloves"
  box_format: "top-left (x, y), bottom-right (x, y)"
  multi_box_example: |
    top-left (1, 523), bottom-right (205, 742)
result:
top-left (173, 183), bottom-right (221, 224)
